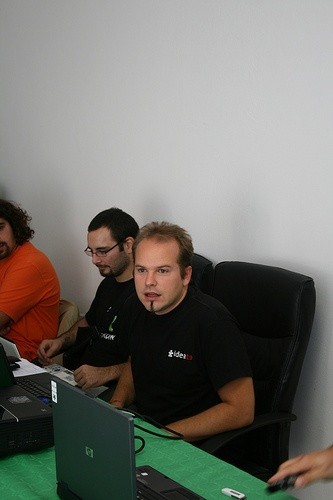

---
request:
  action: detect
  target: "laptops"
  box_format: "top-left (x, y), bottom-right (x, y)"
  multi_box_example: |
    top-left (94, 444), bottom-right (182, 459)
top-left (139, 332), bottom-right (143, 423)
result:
top-left (0, 343), bottom-right (87, 410)
top-left (51, 378), bottom-right (207, 500)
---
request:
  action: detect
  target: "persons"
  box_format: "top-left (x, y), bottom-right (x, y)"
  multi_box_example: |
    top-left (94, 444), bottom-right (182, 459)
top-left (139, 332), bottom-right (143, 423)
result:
top-left (108, 221), bottom-right (254, 447)
top-left (0, 198), bottom-right (60, 362)
top-left (267, 444), bottom-right (333, 489)
top-left (37, 208), bottom-right (140, 389)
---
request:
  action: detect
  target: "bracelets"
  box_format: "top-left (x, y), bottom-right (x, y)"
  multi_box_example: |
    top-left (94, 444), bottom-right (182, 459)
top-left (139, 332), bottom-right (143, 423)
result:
top-left (108, 400), bottom-right (121, 403)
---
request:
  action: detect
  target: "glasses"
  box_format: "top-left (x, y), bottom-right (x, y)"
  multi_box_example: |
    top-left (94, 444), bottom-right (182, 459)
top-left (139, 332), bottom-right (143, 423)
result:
top-left (84, 235), bottom-right (131, 257)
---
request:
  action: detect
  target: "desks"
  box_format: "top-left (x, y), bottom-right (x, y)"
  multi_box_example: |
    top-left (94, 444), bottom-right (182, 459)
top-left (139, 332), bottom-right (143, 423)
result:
top-left (0, 358), bottom-right (298, 500)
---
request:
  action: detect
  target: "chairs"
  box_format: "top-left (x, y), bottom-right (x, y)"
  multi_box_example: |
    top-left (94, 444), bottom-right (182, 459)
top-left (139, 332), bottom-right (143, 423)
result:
top-left (192, 254), bottom-right (316, 482)
